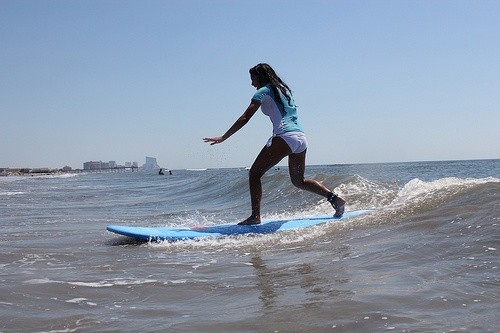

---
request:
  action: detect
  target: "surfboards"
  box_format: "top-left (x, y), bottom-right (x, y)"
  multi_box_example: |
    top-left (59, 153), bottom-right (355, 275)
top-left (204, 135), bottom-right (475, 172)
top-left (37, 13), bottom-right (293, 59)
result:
top-left (107, 209), bottom-right (372, 241)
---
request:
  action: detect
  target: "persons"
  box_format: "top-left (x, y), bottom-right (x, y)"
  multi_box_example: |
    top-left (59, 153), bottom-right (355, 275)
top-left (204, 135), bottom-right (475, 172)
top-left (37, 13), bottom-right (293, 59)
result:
top-left (203, 63), bottom-right (346, 225)
top-left (159, 169), bottom-right (164, 175)
top-left (169, 170), bottom-right (172, 175)
top-left (247, 169), bottom-right (249, 171)
